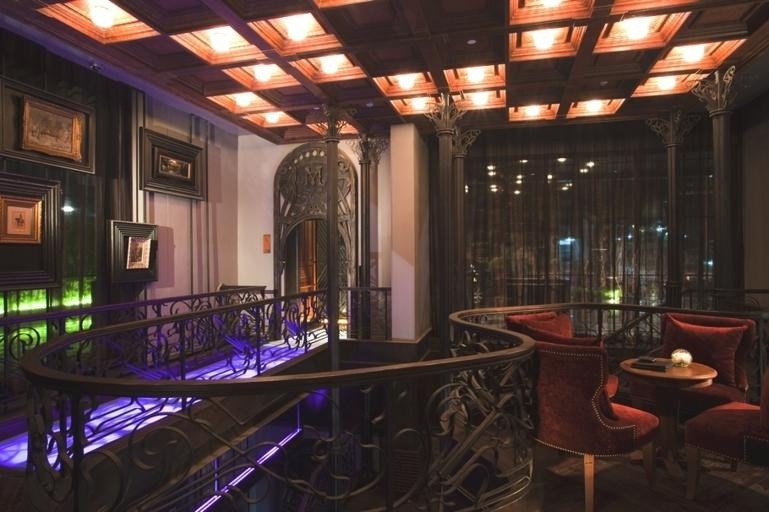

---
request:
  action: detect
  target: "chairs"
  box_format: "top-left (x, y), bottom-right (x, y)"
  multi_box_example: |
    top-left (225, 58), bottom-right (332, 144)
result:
top-left (505, 311), bottom-right (620, 457)
top-left (649, 310), bottom-right (757, 471)
top-left (669, 367), bottom-right (769, 499)
top-left (532, 343), bottom-right (660, 512)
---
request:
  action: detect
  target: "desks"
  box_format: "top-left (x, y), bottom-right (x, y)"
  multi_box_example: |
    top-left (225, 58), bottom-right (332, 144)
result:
top-left (619, 357), bottom-right (718, 492)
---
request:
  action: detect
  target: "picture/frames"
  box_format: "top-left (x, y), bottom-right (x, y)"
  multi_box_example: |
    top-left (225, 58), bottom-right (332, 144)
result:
top-left (0, 195), bottom-right (43, 244)
top-left (20, 94), bottom-right (85, 163)
top-left (126, 236), bottom-right (151, 270)
top-left (153, 146), bottom-right (195, 185)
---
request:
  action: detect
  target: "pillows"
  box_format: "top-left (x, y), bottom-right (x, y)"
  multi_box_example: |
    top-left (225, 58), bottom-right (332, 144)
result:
top-left (661, 314), bottom-right (747, 388)
top-left (520, 319), bottom-right (619, 421)
